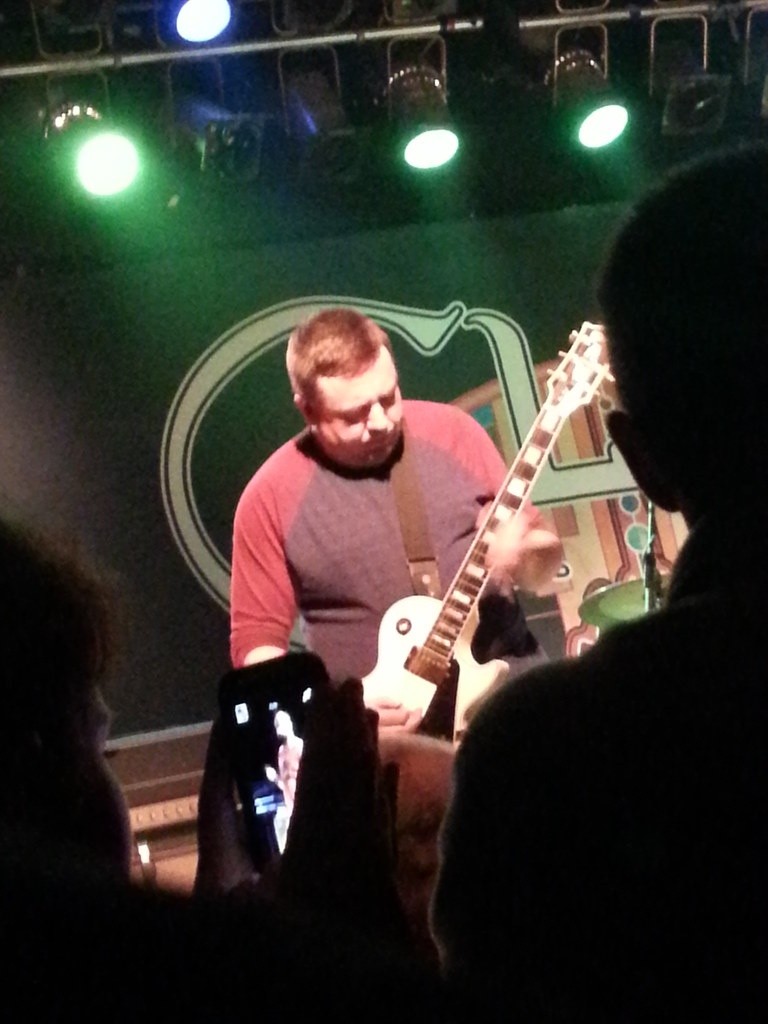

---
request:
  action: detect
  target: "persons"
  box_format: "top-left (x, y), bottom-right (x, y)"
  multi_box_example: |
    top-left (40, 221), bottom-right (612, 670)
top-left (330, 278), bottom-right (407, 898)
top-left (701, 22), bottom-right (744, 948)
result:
top-left (263, 706), bottom-right (304, 819)
top-left (427, 141), bottom-right (765, 1023)
top-left (3, 514), bottom-right (396, 1024)
top-left (231, 309), bottom-right (564, 740)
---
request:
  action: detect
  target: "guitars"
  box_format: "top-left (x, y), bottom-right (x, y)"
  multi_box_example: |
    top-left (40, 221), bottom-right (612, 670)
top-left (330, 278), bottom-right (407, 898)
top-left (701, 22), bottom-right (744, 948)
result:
top-left (362, 320), bottom-right (617, 742)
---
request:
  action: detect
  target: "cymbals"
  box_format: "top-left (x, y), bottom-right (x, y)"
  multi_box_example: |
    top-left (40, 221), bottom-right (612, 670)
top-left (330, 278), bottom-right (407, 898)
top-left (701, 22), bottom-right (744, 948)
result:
top-left (576, 572), bottom-right (673, 628)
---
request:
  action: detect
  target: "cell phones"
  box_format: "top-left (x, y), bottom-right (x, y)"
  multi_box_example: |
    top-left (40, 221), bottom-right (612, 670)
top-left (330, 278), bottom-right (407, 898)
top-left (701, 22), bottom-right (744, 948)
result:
top-left (137, 840), bottom-right (151, 865)
top-left (216, 651), bottom-right (336, 872)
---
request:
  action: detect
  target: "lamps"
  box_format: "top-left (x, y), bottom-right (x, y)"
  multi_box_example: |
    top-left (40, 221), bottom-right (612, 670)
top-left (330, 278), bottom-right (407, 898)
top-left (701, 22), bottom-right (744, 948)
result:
top-left (170, 0), bottom-right (235, 45)
top-left (283, 70), bottom-right (350, 146)
top-left (543, 48), bottom-right (631, 151)
top-left (42, 101), bottom-right (144, 200)
top-left (384, 58), bottom-right (463, 172)
top-left (656, 71), bottom-right (732, 138)
